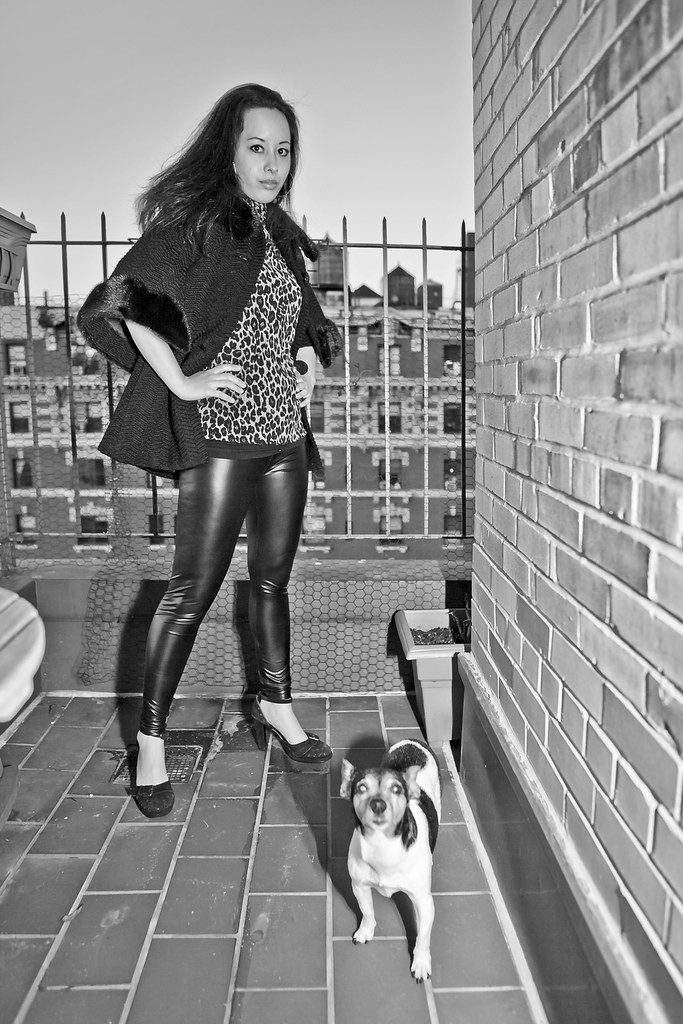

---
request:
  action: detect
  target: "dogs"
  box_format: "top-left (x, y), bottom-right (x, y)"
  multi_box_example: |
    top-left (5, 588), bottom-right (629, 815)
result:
top-left (339, 736), bottom-right (443, 983)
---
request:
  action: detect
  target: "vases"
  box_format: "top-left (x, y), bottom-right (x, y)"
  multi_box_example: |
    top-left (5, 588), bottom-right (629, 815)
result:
top-left (394, 608), bottom-right (472, 743)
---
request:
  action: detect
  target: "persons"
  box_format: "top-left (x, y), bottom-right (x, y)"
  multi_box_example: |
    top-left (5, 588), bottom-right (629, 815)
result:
top-left (77, 83), bottom-right (343, 818)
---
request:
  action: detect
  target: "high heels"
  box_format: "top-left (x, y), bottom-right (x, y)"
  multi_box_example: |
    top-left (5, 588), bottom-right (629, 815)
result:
top-left (251, 695), bottom-right (333, 763)
top-left (132, 741), bottom-right (175, 818)
